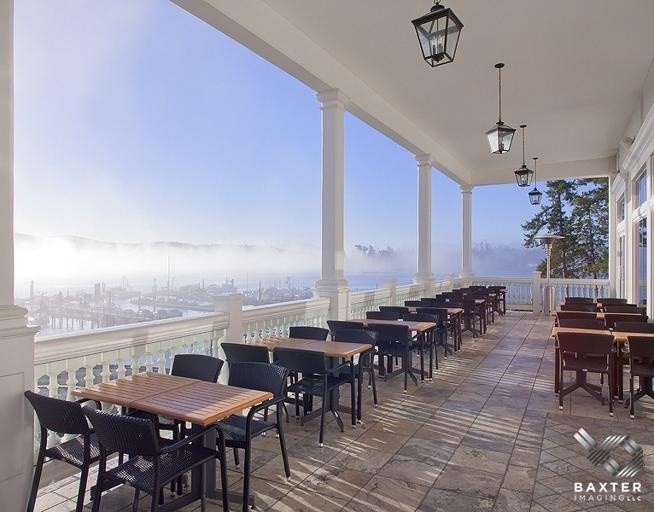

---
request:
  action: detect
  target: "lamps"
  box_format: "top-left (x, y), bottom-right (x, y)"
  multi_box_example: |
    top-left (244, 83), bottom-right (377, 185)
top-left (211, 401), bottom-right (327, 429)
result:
top-left (529, 153), bottom-right (543, 204)
top-left (478, 59), bottom-right (516, 155)
top-left (409, 0), bottom-right (464, 66)
top-left (514, 122), bottom-right (534, 187)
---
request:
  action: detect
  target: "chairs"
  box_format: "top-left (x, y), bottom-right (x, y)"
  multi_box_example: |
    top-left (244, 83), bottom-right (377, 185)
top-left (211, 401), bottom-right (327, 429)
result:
top-left (225, 283), bottom-right (507, 448)
top-left (26, 390), bottom-right (115, 512)
top-left (549, 292), bottom-right (654, 416)
top-left (86, 412), bottom-right (231, 511)
top-left (211, 364), bottom-right (293, 512)
top-left (149, 354), bottom-right (225, 432)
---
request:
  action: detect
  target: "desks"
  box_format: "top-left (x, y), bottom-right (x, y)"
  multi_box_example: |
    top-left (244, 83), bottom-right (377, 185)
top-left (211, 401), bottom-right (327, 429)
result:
top-left (78, 369), bottom-right (273, 512)
